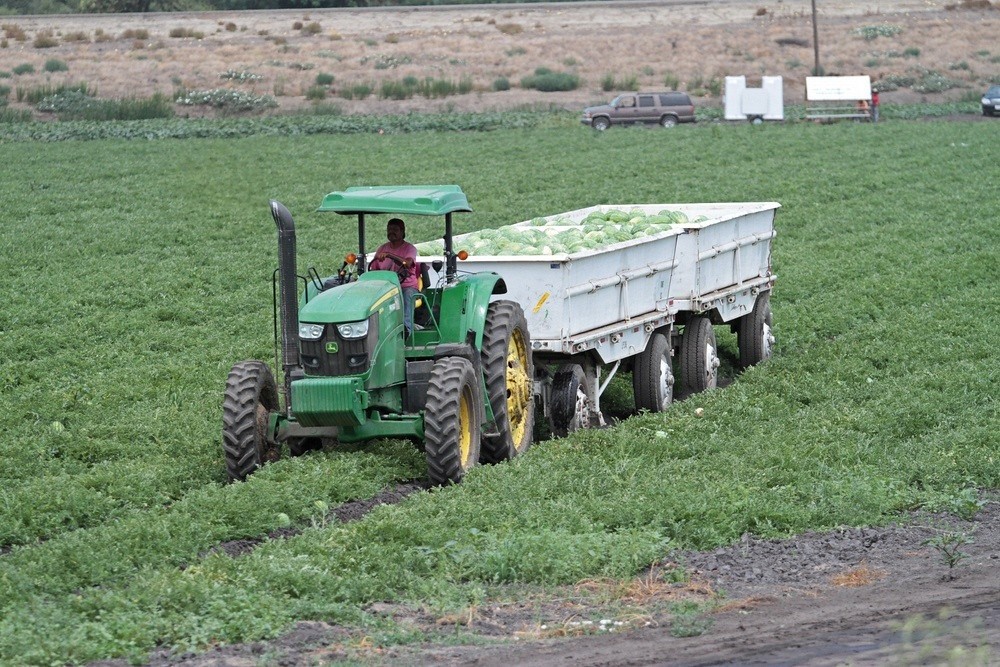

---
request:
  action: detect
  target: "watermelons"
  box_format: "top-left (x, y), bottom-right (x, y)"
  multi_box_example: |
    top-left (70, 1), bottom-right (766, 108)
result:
top-left (274, 499), bottom-right (328, 527)
top-left (418, 208), bottom-right (708, 256)
top-left (50, 421), bottom-right (63, 436)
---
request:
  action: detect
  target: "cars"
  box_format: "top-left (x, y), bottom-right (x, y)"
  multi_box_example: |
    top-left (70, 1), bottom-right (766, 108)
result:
top-left (981, 86), bottom-right (1000, 117)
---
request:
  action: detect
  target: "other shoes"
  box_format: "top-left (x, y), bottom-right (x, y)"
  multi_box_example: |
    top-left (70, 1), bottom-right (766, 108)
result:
top-left (404, 325), bottom-right (409, 343)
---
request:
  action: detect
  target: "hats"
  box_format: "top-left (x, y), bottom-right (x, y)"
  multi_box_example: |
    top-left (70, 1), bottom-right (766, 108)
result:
top-left (388, 218), bottom-right (405, 239)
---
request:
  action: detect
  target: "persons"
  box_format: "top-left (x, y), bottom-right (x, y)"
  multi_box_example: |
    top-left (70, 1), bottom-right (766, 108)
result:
top-left (370, 218), bottom-right (419, 345)
top-left (857, 100), bottom-right (870, 122)
top-left (872, 88), bottom-right (879, 122)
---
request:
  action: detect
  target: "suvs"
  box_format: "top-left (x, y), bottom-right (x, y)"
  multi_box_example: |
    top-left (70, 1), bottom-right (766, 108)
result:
top-left (581, 91), bottom-right (695, 132)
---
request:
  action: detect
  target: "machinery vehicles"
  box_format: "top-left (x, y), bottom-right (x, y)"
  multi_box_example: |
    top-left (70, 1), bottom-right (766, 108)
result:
top-left (221, 184), bottom-right (783, 488)
